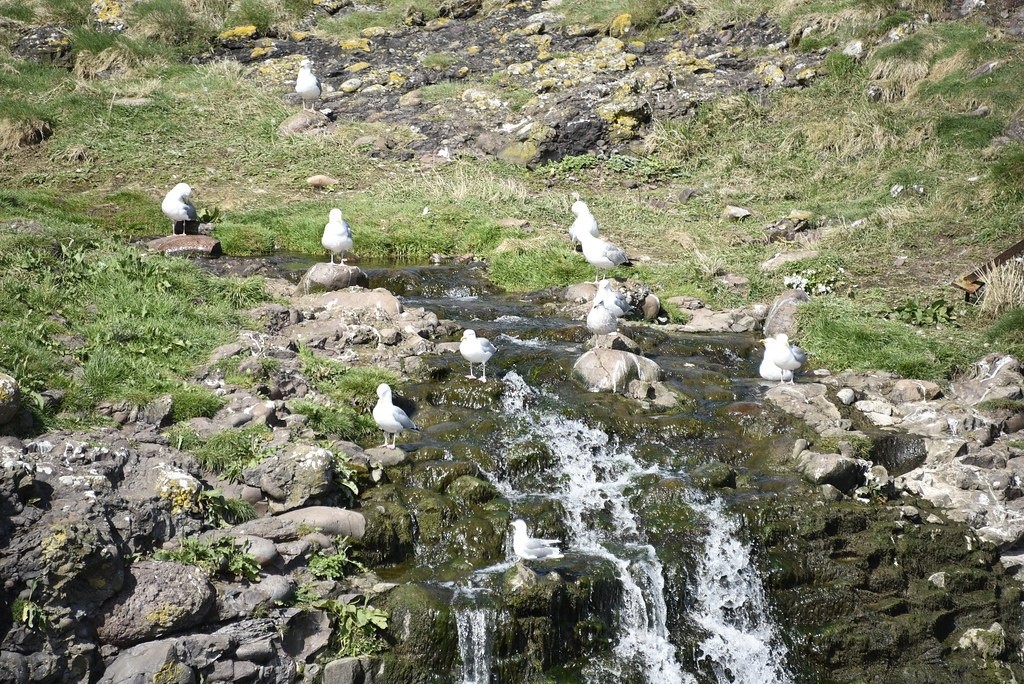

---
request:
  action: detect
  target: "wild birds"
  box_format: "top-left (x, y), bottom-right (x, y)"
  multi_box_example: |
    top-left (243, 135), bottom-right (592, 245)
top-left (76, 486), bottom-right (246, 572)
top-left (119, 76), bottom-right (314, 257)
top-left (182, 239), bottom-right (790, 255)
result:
top-left (373, 383), bottom-right (421, 449)
top-left (759, 333), bottom-right (804, 385)
top-left (587, 280), bottom-right (637, 352)
top-left (162, 183), bottom-right (198, 235)
top-left (321, 208), bottom-right (354, 267)
top-left (295, 60), bottom-right (322, 114)
top-left (570, 200), bottom-right (629, 283)
top-left (459, 329), bottom-right (501, 383)
top-left (510, 518), bottom-right (562, 559)
